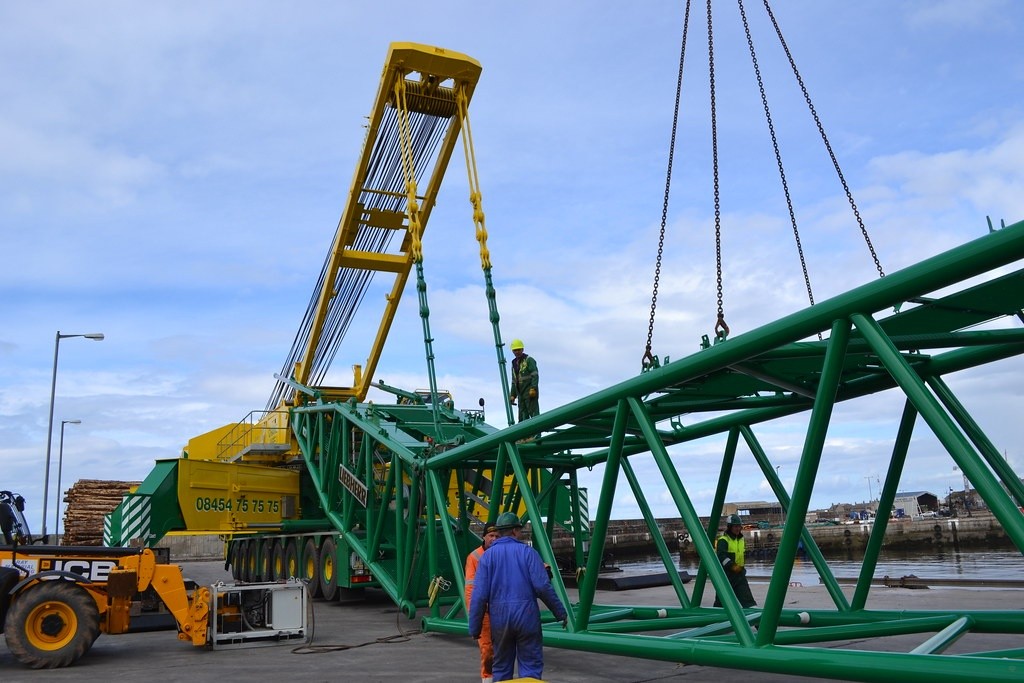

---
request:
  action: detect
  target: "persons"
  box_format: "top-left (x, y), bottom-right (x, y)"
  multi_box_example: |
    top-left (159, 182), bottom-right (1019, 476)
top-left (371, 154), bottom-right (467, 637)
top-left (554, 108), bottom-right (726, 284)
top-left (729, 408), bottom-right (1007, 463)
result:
top-left (511, 339), bottom-right (541, 441)
top-left (468, 512), bottom-right (567, 681)
top-left (713, 514), bottom-right (760, 631)
top-left (465, 521), bottom-right (500, 683)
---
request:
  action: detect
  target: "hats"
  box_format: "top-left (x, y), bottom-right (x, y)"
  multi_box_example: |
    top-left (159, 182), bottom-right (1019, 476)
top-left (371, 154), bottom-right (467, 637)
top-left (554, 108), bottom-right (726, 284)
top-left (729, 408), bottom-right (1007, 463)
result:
top-left (483, 521), bottom-right (498, 536)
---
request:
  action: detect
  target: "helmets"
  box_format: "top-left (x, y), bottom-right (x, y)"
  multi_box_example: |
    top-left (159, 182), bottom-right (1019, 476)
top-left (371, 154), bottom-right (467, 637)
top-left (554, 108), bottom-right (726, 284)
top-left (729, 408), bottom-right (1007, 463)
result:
top-left (727, 514), bottom-right (744, 524)
top-left (511, 339), bottom-right (524, 351)
top-left (495, 512), bottom-right (522, 530)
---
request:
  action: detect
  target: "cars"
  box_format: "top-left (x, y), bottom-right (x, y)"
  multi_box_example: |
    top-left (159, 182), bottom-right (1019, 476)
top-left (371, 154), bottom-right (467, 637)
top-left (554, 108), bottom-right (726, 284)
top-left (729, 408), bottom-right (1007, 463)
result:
top-left (840, 519), bottom-right (855, 526)
top-left (815, 518), bottom-right (830, 523)
top-left (920, 511), bottom-right (938, 518)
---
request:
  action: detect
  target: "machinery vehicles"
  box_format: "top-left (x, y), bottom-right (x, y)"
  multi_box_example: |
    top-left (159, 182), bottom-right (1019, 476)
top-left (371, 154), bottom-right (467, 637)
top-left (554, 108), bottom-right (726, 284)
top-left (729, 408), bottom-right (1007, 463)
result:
top-left (0, 490), bottom-right (245, 671)
top-left (104, 44), bottom-right (582, 609)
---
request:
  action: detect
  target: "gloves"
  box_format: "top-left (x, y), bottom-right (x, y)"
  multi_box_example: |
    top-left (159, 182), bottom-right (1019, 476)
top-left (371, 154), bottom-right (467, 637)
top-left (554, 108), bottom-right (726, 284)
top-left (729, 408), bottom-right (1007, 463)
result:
top-left (732, 564), bottom-right (741, 573)
top-left (529, 389), bottom-right (536, 397)
top-left (471, 635), bottom-right (481, 640)
top-left (562, 618), bottom-right (567, 629)
top-left (546, 566), bottom-right (553, 581)
top-left (510, 395), bottom-right (517, 405)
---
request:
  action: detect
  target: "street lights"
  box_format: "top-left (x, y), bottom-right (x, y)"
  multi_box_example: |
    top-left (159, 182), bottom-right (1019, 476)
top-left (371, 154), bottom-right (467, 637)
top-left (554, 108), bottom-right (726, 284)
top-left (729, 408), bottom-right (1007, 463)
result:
top-left (54, 419), bottom-right (83, 545)
top-left (776, 465), bottom-right (784, 525)
top-left (43, 331), bottom-right (105, 546)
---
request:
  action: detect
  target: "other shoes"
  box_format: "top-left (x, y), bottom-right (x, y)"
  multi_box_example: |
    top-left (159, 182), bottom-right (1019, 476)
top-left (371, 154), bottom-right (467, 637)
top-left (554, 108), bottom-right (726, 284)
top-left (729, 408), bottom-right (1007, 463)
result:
top-left (515, 437), bottom-right (534, 444)
